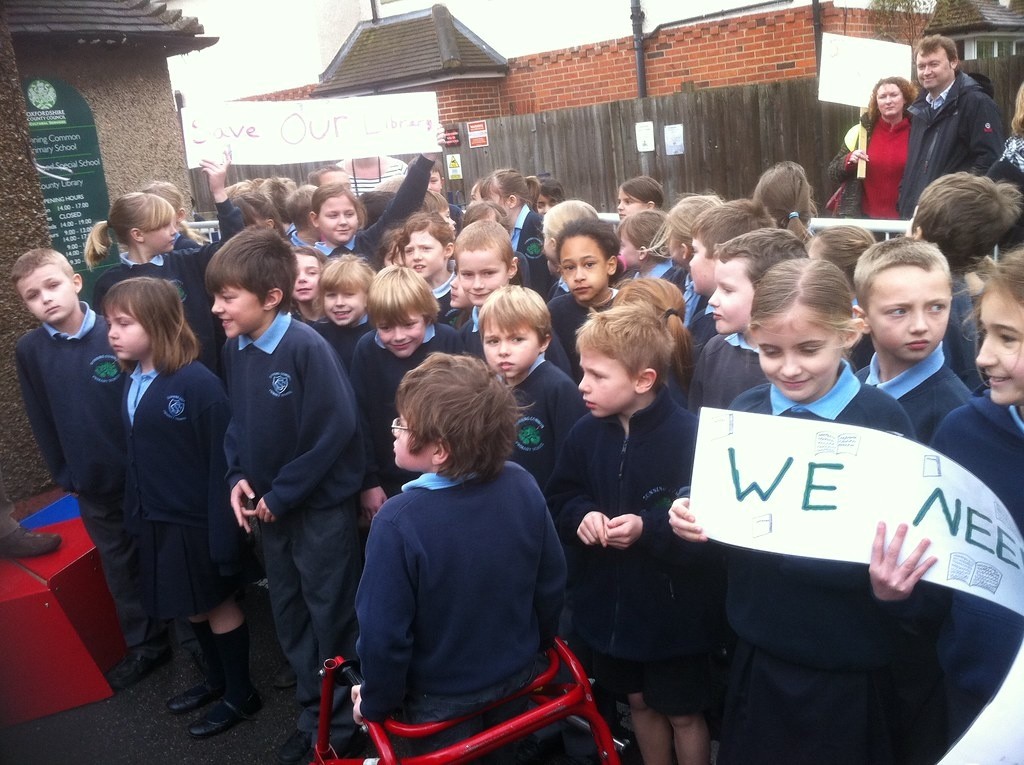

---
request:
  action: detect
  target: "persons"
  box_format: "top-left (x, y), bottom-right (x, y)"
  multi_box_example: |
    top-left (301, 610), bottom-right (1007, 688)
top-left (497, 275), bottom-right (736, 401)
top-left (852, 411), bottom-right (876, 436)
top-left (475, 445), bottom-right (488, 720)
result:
top-left (827, 76), bottom-right (917, 220)
top-left (986, 82), bottom-right (1024, 243)
top-left (10, 124), bottom-right (1024, 765)
top-left (0, 471), bottom-right (60, 556)
top-left (204, 225), bottom-right (367, 765)
top-left (898, 34), bottom-right (1004, 220)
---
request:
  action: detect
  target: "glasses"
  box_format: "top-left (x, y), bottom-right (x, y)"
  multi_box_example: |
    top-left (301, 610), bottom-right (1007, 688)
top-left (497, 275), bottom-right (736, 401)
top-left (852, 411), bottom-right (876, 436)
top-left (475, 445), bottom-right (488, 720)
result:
top-left (390, 417), bottom-right (415, 437)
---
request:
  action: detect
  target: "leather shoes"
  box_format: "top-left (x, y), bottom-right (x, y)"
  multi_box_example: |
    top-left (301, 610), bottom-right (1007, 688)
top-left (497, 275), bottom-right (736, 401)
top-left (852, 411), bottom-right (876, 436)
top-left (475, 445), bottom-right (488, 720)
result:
top-left (166, 680), bottom-right (226, 714)
top-left (0, 524), bottom-right (63, 556)
top-left (187, 704), bottom-right (253, 737)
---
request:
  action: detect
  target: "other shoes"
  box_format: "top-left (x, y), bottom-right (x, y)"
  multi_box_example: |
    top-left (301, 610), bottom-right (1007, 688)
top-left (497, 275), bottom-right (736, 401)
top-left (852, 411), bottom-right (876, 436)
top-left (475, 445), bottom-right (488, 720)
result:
top-left (275, 728), bottom-right (315, 763)
top-left (105, 637), bottom-right (173, 690)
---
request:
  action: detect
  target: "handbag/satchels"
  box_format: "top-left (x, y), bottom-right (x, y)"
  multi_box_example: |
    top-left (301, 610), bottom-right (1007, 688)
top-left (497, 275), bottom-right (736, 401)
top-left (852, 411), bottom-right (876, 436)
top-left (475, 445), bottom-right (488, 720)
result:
top-left (824, 180), bottom-right (846, 210)
